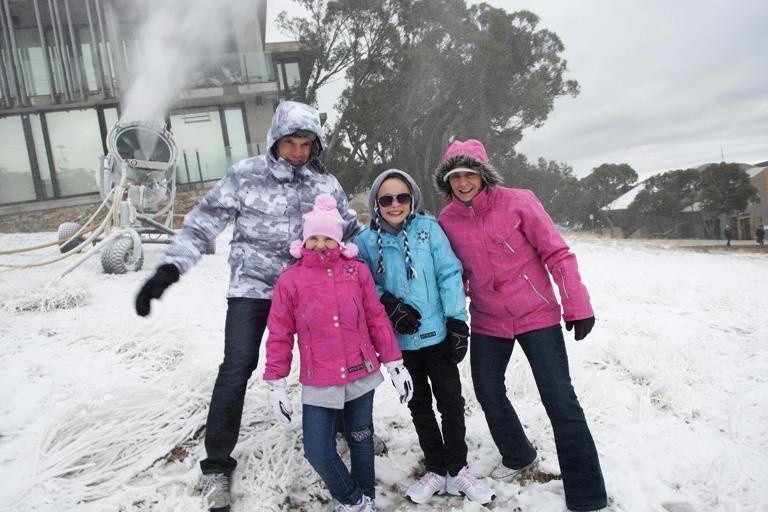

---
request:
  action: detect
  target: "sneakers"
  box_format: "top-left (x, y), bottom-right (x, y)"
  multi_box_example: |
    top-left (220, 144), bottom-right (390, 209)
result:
top-left (372, 434), bottom-right (388, 456)
top-left (404, 471), bottom-right (447, 504)
top-left (490, 455), bottom-right (538, 482)
top-left (202, 473), bottom-right (231, 512)
top-left (331, 493), bottom-right (373, 512)
top-left (446, 464), bottom-right (497, 505)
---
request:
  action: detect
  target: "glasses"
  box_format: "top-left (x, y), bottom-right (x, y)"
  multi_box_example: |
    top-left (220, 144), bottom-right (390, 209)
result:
top-left (376, 193), bottom-right (412, 207)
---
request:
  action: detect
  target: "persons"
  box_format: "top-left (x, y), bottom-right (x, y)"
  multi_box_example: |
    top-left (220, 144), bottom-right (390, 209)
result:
top-left (263, 194), bottom-right (413, 512)
top-left (435, 139), bottom-right (606, 512)
top-left (724, 225), bottom-right (732, 247)
top-left (757, 224), bottom-right (766, 246)
top-left (137, 99), bottom-right (386, 512)
top-left (353, 168), bottom-right (496, 507)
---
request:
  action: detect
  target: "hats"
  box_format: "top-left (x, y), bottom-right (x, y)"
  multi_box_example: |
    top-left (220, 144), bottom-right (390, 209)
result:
top-left (443, 167), bottom-right (479, 182)
top-left (289, 193), bottom-right (358, 259)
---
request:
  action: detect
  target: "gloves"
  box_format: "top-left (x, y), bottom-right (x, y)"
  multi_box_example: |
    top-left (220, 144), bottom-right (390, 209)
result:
top-left (135, 263), bottom-right (180, 317)
top-left (384, 358), bottom-right (414, 404)
top-left (380, 290), bottom-right (422, 335)
top-left (266, 377), bottom-right (293, 424)
top-left (565, 316), bottom-right (595, 341)
top-left (446, 316), bottom-right (469, 364)
